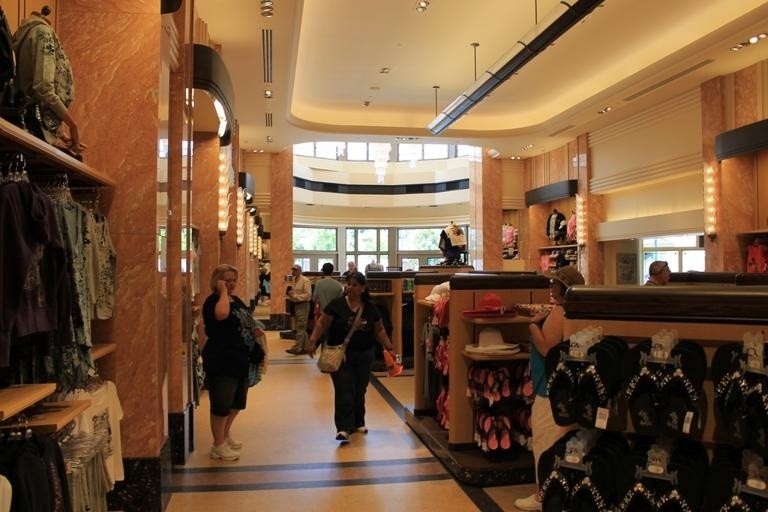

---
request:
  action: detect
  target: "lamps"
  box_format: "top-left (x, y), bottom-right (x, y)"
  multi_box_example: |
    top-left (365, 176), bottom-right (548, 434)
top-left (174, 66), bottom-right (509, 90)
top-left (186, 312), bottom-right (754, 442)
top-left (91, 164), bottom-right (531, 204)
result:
top-left (237, 187), bottom-right (245, 250)
top-left (574, 193), bottom-right (585, 250)
top-left (426, 0), bottom-right (604, 137)
top-left (248, 217), bottom-right (263, 263)
top-left (219, 152), bottom-right (232, 244)
top-left (703, 162), bottom-right (719, 241)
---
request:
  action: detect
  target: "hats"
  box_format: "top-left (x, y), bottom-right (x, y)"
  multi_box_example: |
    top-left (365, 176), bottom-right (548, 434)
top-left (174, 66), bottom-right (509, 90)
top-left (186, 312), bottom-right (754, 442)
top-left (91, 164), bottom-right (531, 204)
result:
top-left (543, 266), bottom-right (584, 287)
top-left (465, 327), bottom-right (520, 355)
top-left (425, 283), bottom-right (449, 301)
top-left (291, 265), bottom-right (300, 270)
top-left (321, 263), bottom-right (333, 271)
top-left (462, 293), bottom-right (516, 319)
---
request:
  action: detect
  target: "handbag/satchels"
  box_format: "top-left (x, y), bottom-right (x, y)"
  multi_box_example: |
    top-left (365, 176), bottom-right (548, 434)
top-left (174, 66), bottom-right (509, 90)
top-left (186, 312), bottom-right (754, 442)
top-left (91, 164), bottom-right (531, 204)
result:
top-left (318, 346), bottom-right (344, 372)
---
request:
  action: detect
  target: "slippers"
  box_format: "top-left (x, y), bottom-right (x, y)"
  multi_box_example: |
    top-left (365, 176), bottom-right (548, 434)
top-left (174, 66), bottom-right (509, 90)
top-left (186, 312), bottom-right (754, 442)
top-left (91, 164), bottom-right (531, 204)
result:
top-left (467, 363), bottom-right (534, 451)
top-left (430, 297), bottom-right (450, 429)
top-left (536, 340), bottom-right (768, 511)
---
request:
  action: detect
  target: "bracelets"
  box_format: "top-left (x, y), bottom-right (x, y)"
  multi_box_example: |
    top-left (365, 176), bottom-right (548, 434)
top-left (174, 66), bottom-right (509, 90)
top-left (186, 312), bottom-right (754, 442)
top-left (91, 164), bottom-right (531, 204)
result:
top-left (386, 348), bottom-right (394, 351)
top-left (529, 321), bottom-right (539, 326)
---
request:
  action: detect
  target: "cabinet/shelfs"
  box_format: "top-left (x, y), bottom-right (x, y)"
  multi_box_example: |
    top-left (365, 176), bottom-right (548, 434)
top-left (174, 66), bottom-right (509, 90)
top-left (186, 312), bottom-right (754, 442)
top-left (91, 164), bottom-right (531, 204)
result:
top-left (503, 249), bottom-right (519, 254)
top-left (540, 244), bottom-right (577, 250)
top-left (0, 119), bottom-right (118, 430)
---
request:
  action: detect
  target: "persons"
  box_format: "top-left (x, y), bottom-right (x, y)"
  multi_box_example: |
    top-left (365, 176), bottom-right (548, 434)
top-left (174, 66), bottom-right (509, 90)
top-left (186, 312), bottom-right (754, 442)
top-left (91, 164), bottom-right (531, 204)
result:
top-left (11, 5), bottom-right (88, 154)
top-left (202, 264), bottom-right (255, 461)
top-left (259, 269), bottom-right (269, 306)
top-left (343, 261), bottom-right (358, 276)
top-left (306, 272), bottom-right (393, 441)
top-left (312, 263), bottom-right (342, 373)
top-left (515, 266), bottom-right (584, 511)
top-left (285, 265), bottom-right (312, 355)
top-left (645, 261), bottom-right (671, 285)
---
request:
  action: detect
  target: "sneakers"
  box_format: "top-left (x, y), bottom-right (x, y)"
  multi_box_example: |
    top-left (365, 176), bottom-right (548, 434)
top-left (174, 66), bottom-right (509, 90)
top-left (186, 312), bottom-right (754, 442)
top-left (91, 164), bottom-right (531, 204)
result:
top-left (285, 347), bottom-right (307, 355)
top-left (210, 437), bottom-right (241, 460)
top-left (514, 495), bottom-right (541, 512)
top-left (336, 426), bottom-right (368, 439)
